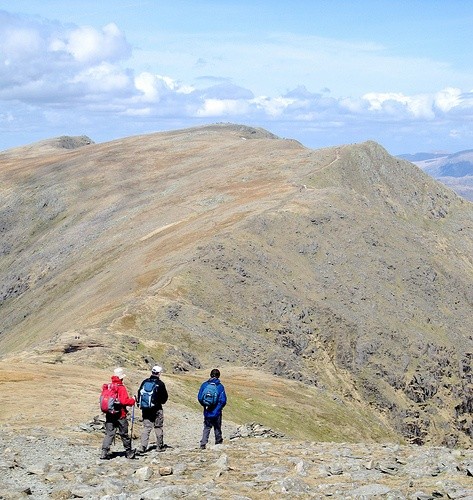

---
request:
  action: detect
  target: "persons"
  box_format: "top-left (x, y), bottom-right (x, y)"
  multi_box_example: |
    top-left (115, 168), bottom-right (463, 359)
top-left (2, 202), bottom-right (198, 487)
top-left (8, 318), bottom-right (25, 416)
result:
top-left (99, 368), bottom-right (138, 459)
top-left (198, 369), bottom-right (228, 449)
top-left (137, 366), bottom-right (169, 452)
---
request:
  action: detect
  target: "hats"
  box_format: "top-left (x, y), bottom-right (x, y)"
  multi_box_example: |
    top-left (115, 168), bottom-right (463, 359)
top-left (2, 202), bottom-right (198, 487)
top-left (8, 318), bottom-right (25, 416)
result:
top-left (152, 366), bottom-right (162, 372)
top-left (112, 368), bottom-right (126, 379)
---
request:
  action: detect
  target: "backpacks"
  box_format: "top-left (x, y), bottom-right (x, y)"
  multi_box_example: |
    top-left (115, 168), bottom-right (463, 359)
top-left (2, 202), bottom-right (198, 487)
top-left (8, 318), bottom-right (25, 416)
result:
top-left (100, 383), bottom-right (125, 413)
top-left (139, 378), bottom-right (158, 408)
top-left (201, 381), bottom-right (219, 407)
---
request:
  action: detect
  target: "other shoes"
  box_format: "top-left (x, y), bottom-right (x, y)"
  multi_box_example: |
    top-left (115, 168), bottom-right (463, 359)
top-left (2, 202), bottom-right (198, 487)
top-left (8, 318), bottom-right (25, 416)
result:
top-left (200, 444), bottom-right (205, 449)
top-left (158, 444), bottom-right (165, 451)
top-left (141, 446), bottom-right (146, 452)
top-left (125, 450), bottom-right (135, 457)
top-left (100, 453), bottom-right (112, 459)
